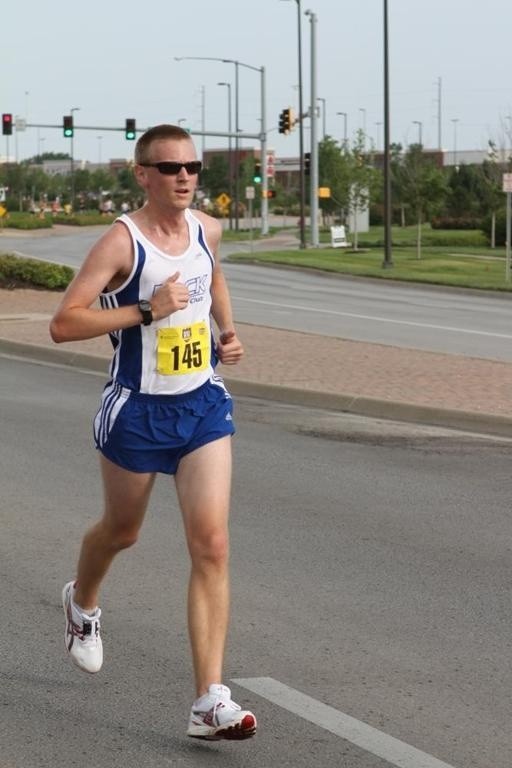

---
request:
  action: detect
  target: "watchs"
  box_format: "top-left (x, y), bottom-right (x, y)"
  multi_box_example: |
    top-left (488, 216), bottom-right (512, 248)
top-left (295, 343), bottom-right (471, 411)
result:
top-left (138, 299), bottom-right (153, 325)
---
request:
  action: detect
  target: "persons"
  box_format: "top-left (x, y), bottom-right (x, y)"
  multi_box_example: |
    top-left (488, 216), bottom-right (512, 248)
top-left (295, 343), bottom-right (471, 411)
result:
top-left (30, 198), bottom-right (129, 220)
top-left (49, 124), bottom-right (258, 740)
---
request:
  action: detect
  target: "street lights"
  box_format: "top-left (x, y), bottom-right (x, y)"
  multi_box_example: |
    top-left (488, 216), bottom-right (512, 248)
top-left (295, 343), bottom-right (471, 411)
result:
top-left (316, 94), bottom-right (462, 233)
top-left (174, 54), bottom-right (271, 238)
top-left (68, 105), bottom-right (80, 215)
top-left (216, 80), bottom-right (233, 230)
top-left (177, 118), bottom-right (187, 128)
top-left (97, 135), bottom-right (103, 197)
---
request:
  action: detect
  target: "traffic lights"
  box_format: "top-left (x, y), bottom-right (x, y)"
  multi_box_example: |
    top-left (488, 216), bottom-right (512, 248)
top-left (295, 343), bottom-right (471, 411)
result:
top-left (2, 112), bottom-right (12, 136)
top-left (267, 189), bottom-right (277, 200)
top-left (253, 163), bottom-right (261, 184)
top-left (63, 115), bottom-right (73, 138)
top-left (124, 117), bottom-right (135, 140)
top-left (278, 107), bottom-right (294, 135)
top-left (304, 150), bottom-right (312, 176)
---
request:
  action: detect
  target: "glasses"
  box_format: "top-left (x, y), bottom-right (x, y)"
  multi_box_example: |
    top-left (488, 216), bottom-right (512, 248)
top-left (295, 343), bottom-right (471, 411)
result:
top-left (138, 161), bottom-right (202, 175)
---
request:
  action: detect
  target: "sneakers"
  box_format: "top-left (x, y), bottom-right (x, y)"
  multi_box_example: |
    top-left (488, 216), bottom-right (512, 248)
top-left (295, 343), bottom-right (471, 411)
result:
top-left (186, 683), bottom-right (257, 741)
top-left (62, 580), bottom-right (104, 673)
